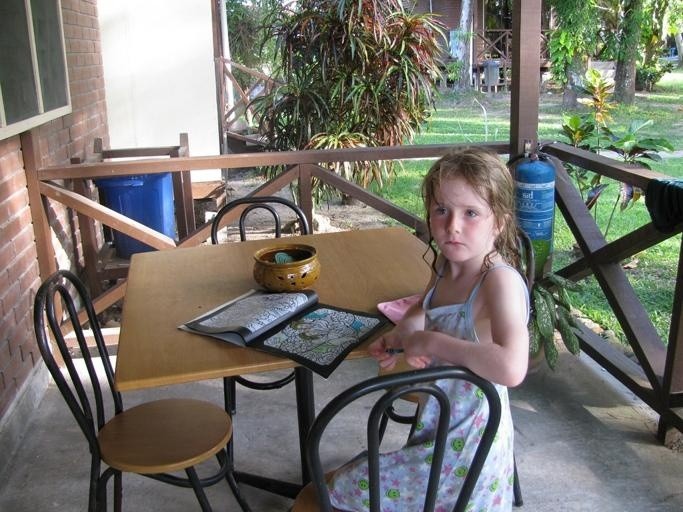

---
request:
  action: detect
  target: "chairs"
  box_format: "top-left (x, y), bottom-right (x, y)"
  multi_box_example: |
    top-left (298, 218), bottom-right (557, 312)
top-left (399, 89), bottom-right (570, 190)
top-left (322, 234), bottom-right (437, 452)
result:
top-left (305, 364), bottom-right (503, 511)
top-left (33, 270), bottom-right (251, 511)
top-left (212, 195), bottom-right (313, 472)
top-left (376, 228), bottom-right (539, 506)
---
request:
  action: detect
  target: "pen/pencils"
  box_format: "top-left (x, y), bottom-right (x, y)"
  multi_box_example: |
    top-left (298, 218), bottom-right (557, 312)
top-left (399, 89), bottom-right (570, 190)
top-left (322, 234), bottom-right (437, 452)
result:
top-left (385, 348), bottom-right (404, 354)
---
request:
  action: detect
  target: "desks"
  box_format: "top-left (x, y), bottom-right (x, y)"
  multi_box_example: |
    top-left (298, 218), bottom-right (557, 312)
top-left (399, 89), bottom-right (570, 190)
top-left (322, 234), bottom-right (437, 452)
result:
top-left (111, 227), bottom-right (438, 507)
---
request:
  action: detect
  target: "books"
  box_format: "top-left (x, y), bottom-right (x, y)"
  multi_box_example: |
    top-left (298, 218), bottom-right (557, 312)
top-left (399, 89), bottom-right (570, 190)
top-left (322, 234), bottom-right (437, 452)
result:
top-left (178, 289), bottom-right (390, 379)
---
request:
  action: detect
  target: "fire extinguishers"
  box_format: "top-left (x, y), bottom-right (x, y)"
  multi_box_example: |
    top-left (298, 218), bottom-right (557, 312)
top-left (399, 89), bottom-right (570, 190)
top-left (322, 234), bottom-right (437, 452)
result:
top-left (505, 139), bottom-right (555, 255)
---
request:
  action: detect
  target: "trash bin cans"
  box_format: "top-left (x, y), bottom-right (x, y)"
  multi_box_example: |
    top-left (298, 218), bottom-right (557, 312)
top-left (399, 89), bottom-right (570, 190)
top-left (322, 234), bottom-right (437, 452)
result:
top-left (483, 60), bottom-right (499, 93)
top-left (92, 172), bottom-right (176, 260)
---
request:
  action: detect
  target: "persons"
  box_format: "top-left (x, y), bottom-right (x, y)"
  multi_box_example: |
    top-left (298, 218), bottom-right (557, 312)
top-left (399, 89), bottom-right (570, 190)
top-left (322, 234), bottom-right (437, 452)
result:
top-left (289, 146), bottom-right (530, 512)
